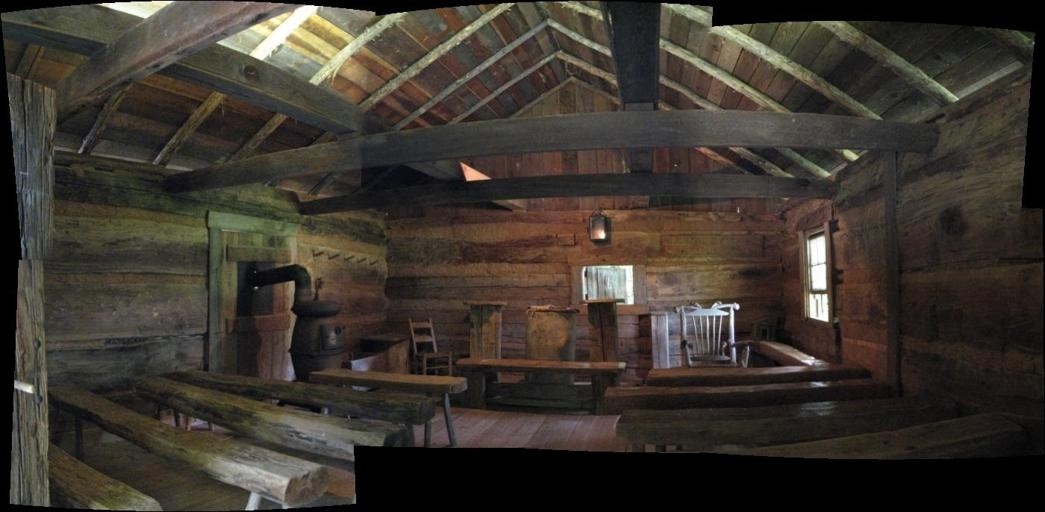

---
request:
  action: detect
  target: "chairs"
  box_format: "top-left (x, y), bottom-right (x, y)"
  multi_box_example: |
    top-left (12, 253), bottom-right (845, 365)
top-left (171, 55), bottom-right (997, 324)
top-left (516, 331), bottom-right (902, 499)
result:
top-left (408, 317), bottom-right (453, 377)
top-left (673, 299), bottom-right (755, 369)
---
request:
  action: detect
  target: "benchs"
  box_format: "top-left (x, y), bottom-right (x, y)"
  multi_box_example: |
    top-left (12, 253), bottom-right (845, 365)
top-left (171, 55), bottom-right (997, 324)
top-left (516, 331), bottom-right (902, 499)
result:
top-left (455, 358), bottom-right (627, 415)
top-left (605, 362), bottom-right (1026, 459)
top-left (47, 368), bottom-right (468, 511)
